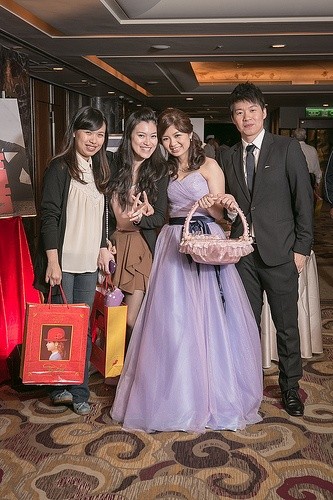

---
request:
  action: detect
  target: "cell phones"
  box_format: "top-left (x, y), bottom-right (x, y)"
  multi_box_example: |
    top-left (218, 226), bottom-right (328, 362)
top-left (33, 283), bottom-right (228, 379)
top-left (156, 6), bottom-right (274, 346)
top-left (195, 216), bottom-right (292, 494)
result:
top-left (109, 260), bottom-right (117, 273)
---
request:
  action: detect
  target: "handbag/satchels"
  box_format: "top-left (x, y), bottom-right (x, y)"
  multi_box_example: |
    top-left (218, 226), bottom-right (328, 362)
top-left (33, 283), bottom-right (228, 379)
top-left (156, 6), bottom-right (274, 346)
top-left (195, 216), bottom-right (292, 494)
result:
top-left (313, 186), bottom-right (323, 217)
top-left (89, 273), bottom-right (128, 378)
top-left (20, 282), bottom-right (89, 386)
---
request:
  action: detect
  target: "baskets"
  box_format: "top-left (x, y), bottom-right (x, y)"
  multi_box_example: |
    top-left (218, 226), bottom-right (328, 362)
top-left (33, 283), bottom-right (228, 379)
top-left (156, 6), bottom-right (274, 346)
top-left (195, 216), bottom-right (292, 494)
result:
top-left (179, 196), bottom-right (252, 266)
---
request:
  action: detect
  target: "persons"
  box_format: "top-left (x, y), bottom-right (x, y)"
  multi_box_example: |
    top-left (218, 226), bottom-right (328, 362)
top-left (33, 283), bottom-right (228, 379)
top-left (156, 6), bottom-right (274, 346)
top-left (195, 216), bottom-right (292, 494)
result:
top-left (109, 109), bottom-right (268, 434)
top-left (203, 135), bottom-right (217, 161)
top-left (213, 80), bottom-right (315, 417)
top-left (290, 126), bottom-right (322, 214)
top-left (104, 109), bottom-right (170, 348)
top-left (32, 106), bottom-right (115, 416)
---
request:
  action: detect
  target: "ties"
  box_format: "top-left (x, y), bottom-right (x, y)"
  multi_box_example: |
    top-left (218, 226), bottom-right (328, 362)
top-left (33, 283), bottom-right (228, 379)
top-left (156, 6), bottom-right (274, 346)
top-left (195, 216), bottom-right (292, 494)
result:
top-left (246, 145), bottom-right (256, 195)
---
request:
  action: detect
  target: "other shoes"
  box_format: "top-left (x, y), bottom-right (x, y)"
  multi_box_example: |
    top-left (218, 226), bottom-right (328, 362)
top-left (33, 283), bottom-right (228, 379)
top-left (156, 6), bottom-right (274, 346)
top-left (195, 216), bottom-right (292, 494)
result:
top-left (53, 391), bottom-right (73, 402)
top-left (75, 402), bottom-right (90, 415)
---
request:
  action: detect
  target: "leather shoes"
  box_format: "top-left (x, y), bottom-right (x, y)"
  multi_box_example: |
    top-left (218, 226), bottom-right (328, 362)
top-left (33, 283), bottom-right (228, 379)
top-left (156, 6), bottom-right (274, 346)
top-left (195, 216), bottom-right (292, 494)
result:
top-left (283, 389), bottom-right (304, 416)
